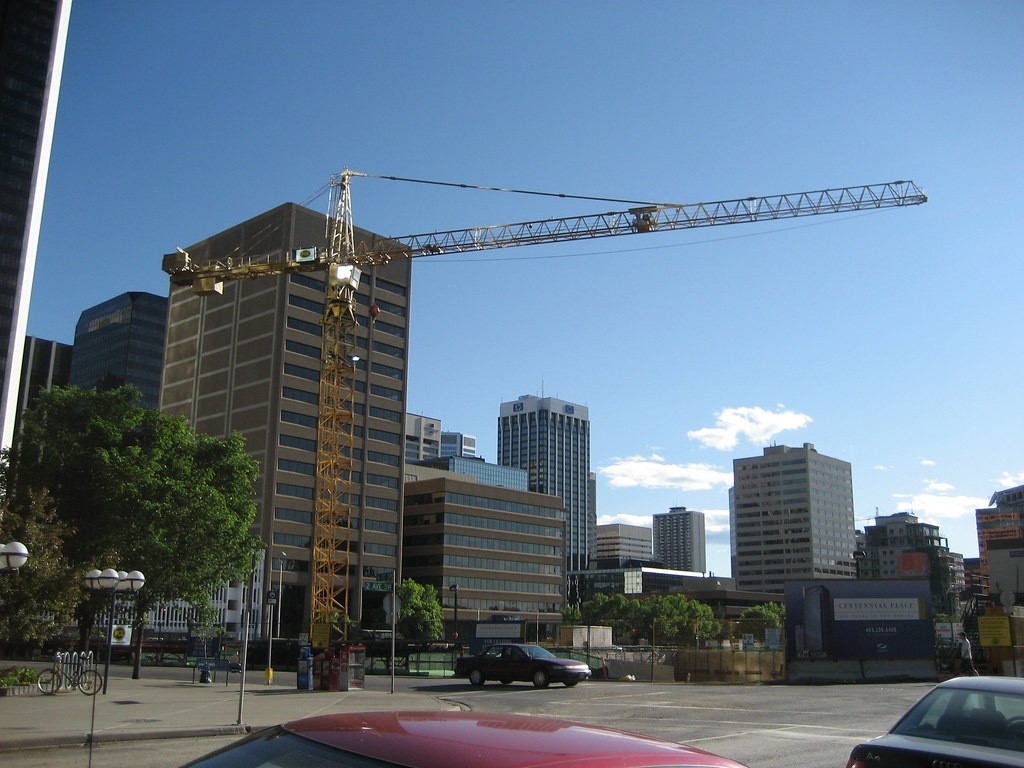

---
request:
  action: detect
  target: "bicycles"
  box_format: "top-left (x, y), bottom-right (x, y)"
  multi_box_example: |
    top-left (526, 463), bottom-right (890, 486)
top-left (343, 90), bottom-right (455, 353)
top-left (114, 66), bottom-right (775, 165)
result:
top-left (37, 651), bottom-right (103, 695)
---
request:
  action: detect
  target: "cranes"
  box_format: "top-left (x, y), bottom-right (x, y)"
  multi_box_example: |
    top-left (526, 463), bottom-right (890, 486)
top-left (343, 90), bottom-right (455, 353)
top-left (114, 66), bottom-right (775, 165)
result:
top-left (161, 164), bottom-right (928, 656)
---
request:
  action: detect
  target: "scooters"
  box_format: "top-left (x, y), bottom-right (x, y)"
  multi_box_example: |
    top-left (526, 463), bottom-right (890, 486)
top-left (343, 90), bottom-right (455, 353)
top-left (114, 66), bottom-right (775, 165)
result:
top-left (201, 669), bottom-right (212, 683)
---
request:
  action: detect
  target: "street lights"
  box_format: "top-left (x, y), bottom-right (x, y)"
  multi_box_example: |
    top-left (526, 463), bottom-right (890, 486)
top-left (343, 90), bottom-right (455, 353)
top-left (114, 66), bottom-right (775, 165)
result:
top-left (449, 583), bottom-right (459, 641)
top-left (83, 568), bottom-right (146, 694)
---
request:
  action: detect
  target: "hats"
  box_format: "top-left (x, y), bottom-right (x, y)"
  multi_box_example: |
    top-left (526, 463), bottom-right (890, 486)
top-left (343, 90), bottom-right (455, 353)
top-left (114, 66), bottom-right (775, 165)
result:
top-left (958, 632), bottom-right (966, 636)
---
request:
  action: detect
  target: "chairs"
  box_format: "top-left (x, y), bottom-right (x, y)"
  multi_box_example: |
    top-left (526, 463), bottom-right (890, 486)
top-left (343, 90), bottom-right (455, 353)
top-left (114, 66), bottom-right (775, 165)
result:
top-left (972, 707), bottom-right (1007, 739)
top-left (937, 711), bottom-right (973, 736)
top-left (501, 651), bottom-right (510, 659)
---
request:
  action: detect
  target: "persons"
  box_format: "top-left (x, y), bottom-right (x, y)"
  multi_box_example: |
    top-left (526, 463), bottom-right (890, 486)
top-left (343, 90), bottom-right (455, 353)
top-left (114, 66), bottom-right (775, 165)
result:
top-left (957, 632), bottom-right (980, 676)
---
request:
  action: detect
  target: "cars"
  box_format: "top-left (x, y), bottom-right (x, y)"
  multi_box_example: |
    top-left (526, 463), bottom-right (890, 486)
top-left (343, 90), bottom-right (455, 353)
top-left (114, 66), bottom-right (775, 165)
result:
top-left (198, 658), bottom-right (216, 670)
top-left (177, 712), bottom-right (751, 768)
top-left (186, 659), bottom-right (197, 667)
top-left (230, 663), bottom-right (242, 673)
top-left (454, 643), bottom-right (592, 688)
top-left (846, 675), bottom-right (1024, 768)
top-left (166, 655), bottom-right (178, 663)
